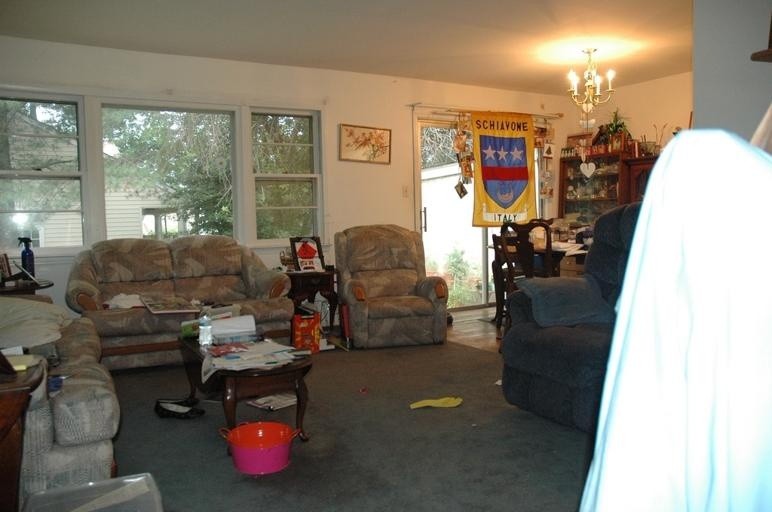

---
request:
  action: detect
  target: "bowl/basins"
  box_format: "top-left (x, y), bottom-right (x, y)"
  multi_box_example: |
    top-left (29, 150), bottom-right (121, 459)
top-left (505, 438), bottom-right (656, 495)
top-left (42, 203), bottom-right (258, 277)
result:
top-left (636, 141), bottom-right (656, 155)
top-left (225, 422), bottom-right (293, 477)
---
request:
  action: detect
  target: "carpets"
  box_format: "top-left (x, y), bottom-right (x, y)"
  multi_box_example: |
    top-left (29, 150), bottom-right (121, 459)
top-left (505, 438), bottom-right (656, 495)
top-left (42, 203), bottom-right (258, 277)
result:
top-left (108, 341), bottom-right (597, 512)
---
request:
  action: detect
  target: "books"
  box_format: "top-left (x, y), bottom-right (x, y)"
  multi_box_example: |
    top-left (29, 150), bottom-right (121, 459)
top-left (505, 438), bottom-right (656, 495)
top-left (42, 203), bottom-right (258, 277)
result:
top-left (139, 292), bottom-right (201, 315)
top-left (246, 392), bottom-right (297, 411)
top-left (5, 354), bottom-right (38, 372)
top-left (550, 241), bottom-right (585, 253)
top-left (210, 332), bottom-right (265, 345)
top-left (565, 250), bottom-right (589, 256)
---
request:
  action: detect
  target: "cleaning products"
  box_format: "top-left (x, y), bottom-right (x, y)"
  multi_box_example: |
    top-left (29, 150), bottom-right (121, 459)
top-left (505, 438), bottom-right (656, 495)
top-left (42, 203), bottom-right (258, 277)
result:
top-left (15, 234), bottom-right (36, 280)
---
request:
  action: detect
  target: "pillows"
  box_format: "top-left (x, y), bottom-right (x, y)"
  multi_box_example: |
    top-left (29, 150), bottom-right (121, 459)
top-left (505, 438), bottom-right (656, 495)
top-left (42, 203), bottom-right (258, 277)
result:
top-left (514, 274), bottom-right (615, 328)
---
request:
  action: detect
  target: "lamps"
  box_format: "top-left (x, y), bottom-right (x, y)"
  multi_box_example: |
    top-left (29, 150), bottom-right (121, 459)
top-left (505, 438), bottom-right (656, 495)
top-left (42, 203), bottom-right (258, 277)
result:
top-left (568, 49), bottom-right (615, 111)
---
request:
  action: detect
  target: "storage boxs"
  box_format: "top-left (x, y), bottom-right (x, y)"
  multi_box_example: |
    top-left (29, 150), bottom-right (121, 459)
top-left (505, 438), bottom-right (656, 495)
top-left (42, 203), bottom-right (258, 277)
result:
top-left (21, 471), bottom-right (162, 512)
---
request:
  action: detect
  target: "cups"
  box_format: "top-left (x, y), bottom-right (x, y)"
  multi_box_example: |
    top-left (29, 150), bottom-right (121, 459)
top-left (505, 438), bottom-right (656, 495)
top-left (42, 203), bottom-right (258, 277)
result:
top-left (559, 144), bottom-right (608, 157)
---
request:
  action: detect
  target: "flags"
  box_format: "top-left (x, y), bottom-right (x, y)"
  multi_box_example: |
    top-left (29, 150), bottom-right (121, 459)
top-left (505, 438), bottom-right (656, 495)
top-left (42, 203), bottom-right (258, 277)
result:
top-left (469, 110), bottom-right (540, 228)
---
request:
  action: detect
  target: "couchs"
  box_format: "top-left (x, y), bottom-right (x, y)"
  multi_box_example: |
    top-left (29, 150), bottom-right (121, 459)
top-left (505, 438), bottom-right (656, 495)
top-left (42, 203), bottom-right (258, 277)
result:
top-left (67, 234), bottom-right (295, 370)
top-left (0, 294), bottom-right (120, 492)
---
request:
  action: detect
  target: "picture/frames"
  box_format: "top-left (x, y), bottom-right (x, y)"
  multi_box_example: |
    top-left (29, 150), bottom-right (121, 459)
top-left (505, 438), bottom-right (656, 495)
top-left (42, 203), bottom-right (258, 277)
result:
top-left (611, 134), bottom-right (624, 153)
top-left (338, 123), bottom-right (392, 166)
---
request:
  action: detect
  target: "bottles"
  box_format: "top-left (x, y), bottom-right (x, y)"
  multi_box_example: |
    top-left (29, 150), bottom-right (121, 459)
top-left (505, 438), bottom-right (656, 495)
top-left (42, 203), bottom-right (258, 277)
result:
top-left (531, 219), bottom-right (582, 246)
top-left (198, 306), bottom-right (213, 346)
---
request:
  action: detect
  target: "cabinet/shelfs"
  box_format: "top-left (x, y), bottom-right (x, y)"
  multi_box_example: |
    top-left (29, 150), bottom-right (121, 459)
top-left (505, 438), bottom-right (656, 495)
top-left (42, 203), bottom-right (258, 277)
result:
top-left (622, 155), bottom-right (661, 209)
top-left (558, 152), bottom-right (630, 217)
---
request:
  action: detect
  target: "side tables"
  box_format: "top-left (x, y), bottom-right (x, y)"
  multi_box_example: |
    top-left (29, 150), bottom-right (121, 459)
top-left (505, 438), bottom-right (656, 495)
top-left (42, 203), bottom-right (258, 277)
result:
top-left (0, 278), bottom-right (54, 295)
top-left (0, 347), bottom-right (44, 512)
top-left (282, 267), bottom-right (339, 330)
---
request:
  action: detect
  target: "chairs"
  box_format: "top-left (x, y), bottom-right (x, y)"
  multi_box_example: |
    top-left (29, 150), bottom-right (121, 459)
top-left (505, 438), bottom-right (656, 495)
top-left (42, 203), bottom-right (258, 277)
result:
top-left (490, 233), bottom-right (523, 338)
top-left (502, 200), bottom-right (646, 430)
top-left (502, 220), bottom-right (557, 292)
top-left (335, 222), bottom-right (449, 350)
